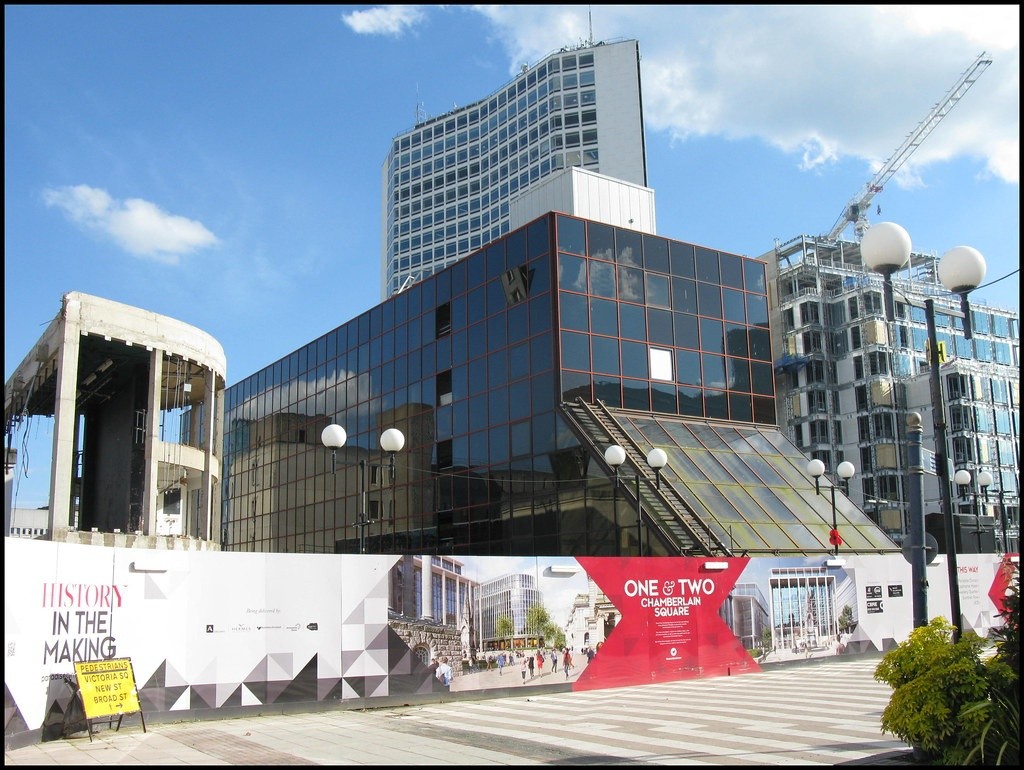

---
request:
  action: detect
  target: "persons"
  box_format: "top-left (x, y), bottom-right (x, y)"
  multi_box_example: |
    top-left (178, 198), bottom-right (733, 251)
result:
top-left (486, 655), bottom-right (494, 671)
top-left (836, 635), bottom-right (846, 655)
top-left (468, 656), bottom-right (480, 674)
top-left (520, 652), bottom-right (527, 684)
top-left (515, 649), bottom-right (525, 658)
top-left (551, 649), bottom-right (558, 673)
top-left (498, 654), bottom-right (504, 676)
top-left (763, 645), bottom-right (766, 660)
top-left (537, 650), bottom-right (542, 678)
top-left (529, 655), bottom-right (534, 677)
top-left (507, 653), bottom-right (514, 666)
top-left (428, 656), bottom-right (452, 692)
top-left (804, 643), bottom-right (808, 658)
top-left (562, 646), bottom-right (575, 680)
top-left (795, 644), bottom-right (797, 654)
top-left (587, 648), bottom-right (594, 664)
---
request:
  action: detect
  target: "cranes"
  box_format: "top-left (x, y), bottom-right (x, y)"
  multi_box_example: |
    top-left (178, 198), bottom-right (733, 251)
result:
top-left (825, 50), bottom-right (993, 245)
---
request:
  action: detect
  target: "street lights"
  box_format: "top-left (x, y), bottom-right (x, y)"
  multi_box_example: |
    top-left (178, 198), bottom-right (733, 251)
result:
top-left (320, 423), bottom-right (405, 554)
top-left (954, 469), bottom-right (993, 554)
top-left (806, 459), bottom-right (856, 553)
top-left (859, 219), bottom-right (985, 649)
top-left (604, 443), bottom-right (667, 557)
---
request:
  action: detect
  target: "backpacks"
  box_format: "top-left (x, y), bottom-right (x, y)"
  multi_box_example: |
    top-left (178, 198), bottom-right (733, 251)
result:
top-left (438, 673), bottom-right (446, 684)
